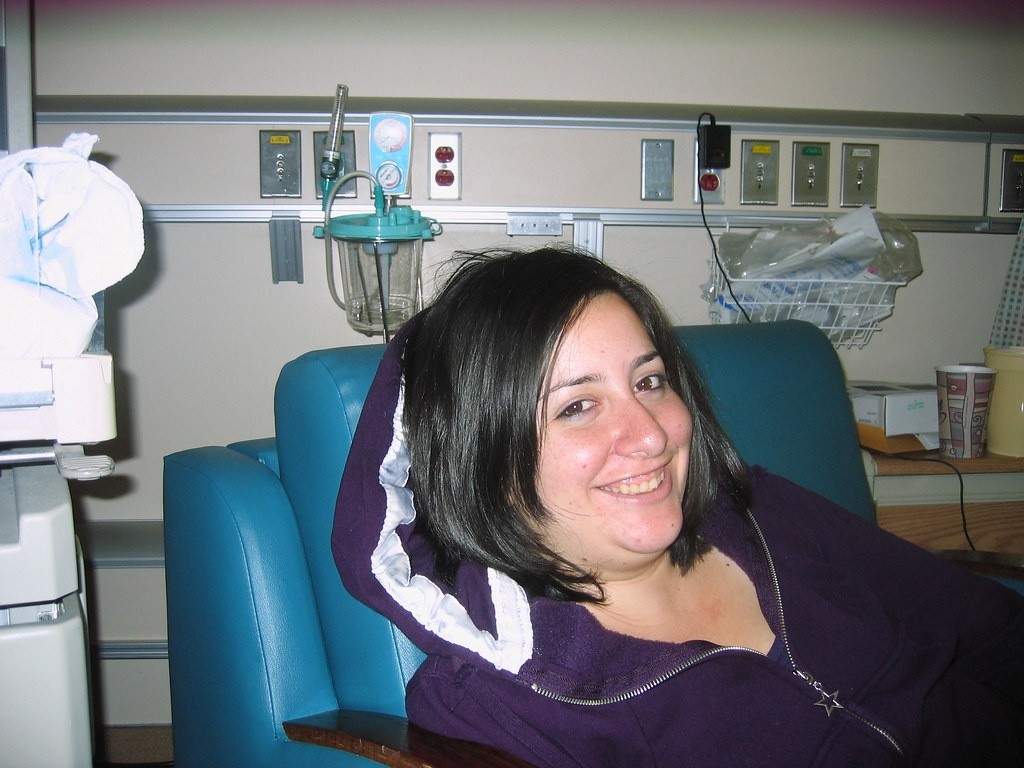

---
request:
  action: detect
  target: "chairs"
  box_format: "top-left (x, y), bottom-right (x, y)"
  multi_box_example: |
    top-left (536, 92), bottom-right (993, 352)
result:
top-left (163, 319), bottom-right (1024, 768)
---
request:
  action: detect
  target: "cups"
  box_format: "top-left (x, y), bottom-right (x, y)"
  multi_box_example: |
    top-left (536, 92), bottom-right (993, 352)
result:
top-left (982, 346), bottom-right (1024, 458)
top-left (935, 366), bottom-right (997, 458)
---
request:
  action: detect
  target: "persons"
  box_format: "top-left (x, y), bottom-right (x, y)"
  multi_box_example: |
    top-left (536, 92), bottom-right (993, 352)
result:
top-left (327, 247), bottom-right (1024, 768)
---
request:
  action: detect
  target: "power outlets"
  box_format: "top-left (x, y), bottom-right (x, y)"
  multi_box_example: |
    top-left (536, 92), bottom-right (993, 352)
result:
top-left (694, 138), bottom-right (725, 204)
top-left (739, 138), bottom-right (780, 206)
top-left (999, 149), bottom-right (1024, 213)
top-left (642, 139), bottom-right (676, 202)
top-left (839, 142), bottom-right (880, 209)
top-left (790, 141), bottom-right (830, 208)
top-left (313, 130), bottom-right (358, 199)
top-left (427, 131), bottom-right (462, 201)
top-left (259, 130), bottom-right (302, 199)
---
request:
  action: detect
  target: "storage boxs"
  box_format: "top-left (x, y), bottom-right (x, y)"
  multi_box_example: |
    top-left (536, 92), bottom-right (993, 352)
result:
top-left (845, 380), bottom-right (939, 436)
top-left (857, 423), bottom-right (926, 454)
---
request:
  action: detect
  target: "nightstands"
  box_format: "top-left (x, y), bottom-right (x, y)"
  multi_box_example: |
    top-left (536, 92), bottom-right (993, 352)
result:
top-left (857, 446), bottom-right (1024, 556)
